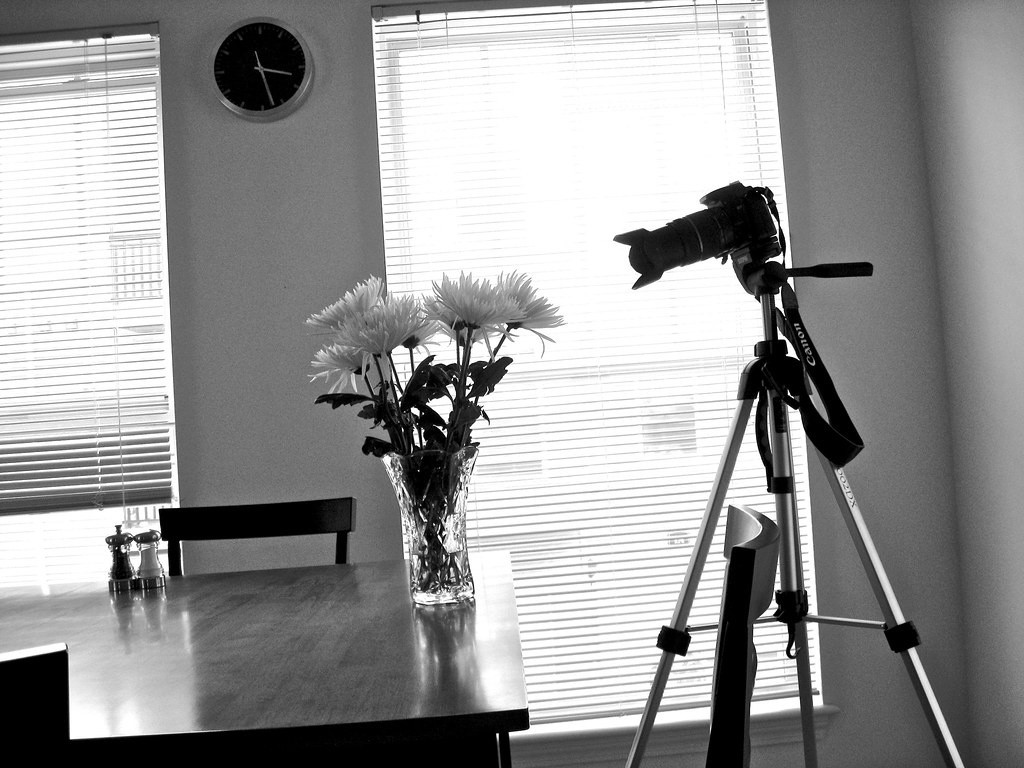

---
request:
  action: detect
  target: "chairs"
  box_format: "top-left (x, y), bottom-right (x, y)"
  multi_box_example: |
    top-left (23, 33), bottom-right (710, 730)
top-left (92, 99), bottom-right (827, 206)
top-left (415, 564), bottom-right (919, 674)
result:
top-left (704, 501), bottom-right (780, 768)
top-left (158, 495), bottom-right (359, 577)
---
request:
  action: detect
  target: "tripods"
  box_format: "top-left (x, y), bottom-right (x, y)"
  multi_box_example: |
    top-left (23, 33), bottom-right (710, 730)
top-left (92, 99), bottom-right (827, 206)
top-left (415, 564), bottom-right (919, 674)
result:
top-left (624, 294), bottom-right (967, 768)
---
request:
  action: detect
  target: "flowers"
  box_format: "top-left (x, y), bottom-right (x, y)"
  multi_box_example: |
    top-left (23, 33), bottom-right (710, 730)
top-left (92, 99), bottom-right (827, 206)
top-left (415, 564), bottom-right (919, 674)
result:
top-left (304, 268), bottom-right (564, 451)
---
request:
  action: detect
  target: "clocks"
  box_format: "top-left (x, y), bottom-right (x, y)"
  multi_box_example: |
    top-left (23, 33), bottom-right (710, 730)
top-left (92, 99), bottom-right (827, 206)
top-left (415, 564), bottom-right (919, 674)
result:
top-left (210, 17), bottom-right (316, 122)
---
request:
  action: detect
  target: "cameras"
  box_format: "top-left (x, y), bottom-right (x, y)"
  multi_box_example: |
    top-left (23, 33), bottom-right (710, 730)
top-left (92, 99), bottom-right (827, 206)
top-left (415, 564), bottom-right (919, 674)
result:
top-left (612, 179), bottom-right (788, 296)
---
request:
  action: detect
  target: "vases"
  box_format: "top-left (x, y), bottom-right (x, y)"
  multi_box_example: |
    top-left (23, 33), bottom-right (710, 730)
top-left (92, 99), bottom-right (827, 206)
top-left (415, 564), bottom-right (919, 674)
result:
top-left (380, 448), bottom-right (477, 605)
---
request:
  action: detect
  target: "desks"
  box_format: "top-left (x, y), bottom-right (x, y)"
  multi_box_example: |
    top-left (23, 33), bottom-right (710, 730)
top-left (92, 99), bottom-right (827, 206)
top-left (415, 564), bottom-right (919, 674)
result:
top-left (0, 550), bottom-right (531, 768)
